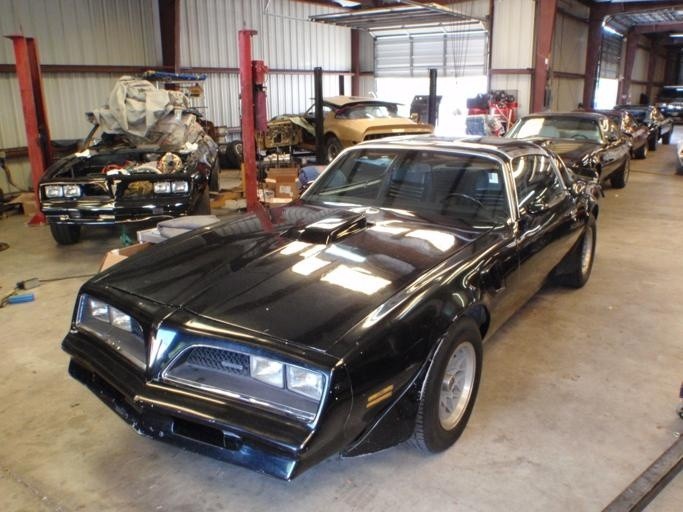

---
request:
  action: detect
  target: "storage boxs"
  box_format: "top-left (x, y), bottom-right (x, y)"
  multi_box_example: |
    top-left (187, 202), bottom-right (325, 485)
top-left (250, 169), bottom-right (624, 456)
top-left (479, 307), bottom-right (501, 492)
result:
top-left (9, 191), bottom-right (37, 216)
top-left (275, 176), bottom-right (297, 198)
top-left (99, 242), bottom-right (152, 273)
top-left (137, 228), bottom-right (167, 243)
top-left (265, 168), bottom-right (297, 190)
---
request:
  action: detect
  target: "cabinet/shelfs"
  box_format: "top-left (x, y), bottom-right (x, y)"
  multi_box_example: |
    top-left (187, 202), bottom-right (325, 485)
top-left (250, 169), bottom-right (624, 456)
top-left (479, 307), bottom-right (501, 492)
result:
top-left (155, 80), bottom-right (206, 134)
top-left (489, 90), bottom-right (518, 128)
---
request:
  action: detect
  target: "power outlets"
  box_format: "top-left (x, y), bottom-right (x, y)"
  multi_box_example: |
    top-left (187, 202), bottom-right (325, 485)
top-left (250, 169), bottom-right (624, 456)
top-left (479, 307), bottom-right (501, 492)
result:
top-left (0, 152), bottom-right (6, 158)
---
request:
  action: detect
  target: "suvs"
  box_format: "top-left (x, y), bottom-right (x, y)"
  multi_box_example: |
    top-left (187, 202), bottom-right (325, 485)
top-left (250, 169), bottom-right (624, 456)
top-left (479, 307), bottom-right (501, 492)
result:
top-left (652, 86), bottom-right (683, 121)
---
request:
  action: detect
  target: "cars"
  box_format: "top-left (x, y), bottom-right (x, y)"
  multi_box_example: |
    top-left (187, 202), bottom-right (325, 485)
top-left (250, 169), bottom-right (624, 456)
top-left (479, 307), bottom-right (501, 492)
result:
top-left (37, 123), bottom-right (221, 245)
top-left (503, 112), bottom-right (633, 189)
top-left (597, 111), bottom-right (651, 159)
top-left (613, 105), bottom-right (674, 151)
top-left (270, 96), bottom-right (433, 163)
top-left (61, 135), bottom-right (602, 481)
